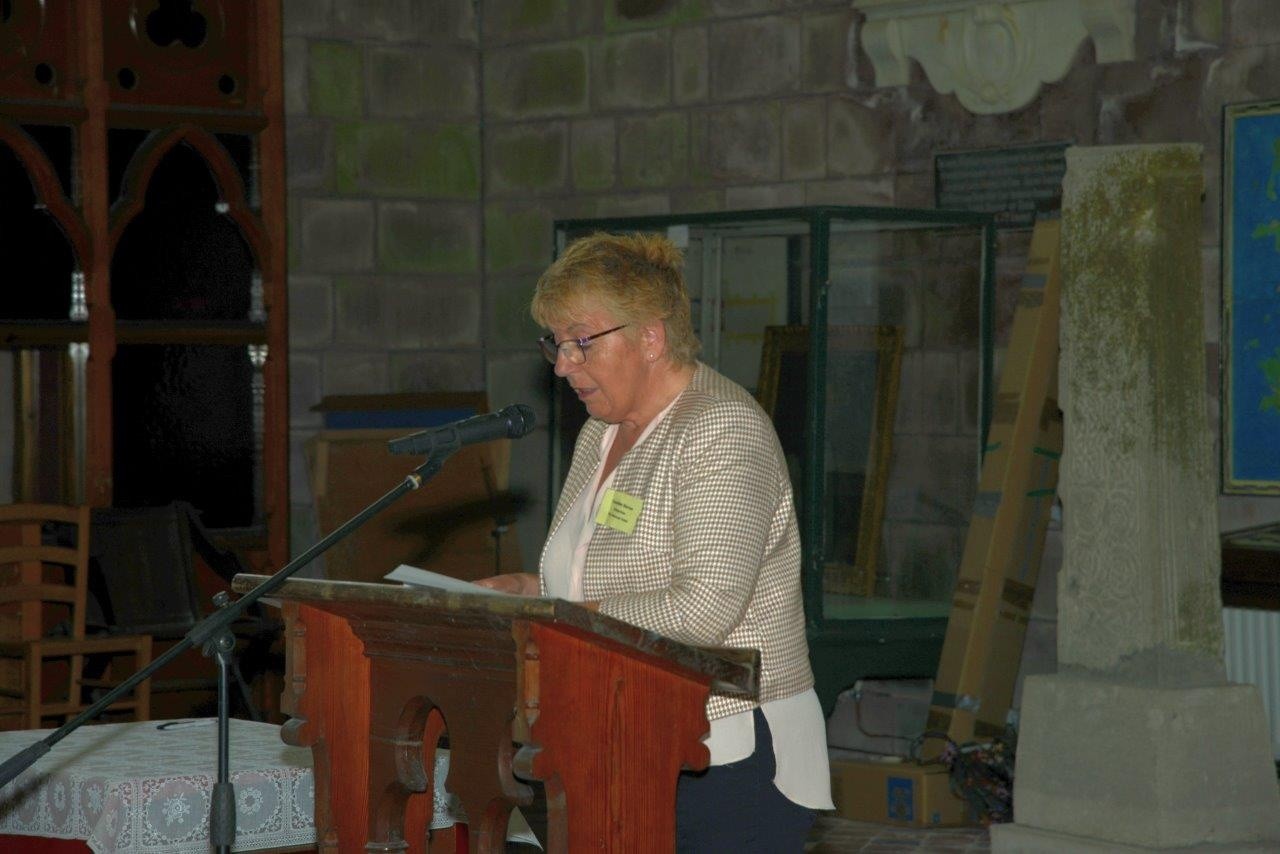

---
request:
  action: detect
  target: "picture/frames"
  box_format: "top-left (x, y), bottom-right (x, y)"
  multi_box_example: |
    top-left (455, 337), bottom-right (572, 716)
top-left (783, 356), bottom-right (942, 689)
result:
top-left (1221, 98), bottom-right (1280, 497)
top-left (758, 321), bottom-right (906, 599)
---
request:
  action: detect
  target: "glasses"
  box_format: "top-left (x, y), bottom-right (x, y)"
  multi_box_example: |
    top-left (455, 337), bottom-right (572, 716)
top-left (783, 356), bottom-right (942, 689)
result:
top-left (535, 324), bottom-right (629, 366)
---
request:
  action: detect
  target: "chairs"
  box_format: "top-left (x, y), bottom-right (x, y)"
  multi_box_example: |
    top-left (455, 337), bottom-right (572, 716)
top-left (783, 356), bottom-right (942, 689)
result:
top-left (0, 504), bottom-right (281, 731)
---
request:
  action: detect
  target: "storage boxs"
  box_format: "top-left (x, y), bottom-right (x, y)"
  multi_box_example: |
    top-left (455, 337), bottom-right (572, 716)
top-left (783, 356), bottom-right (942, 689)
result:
top-left (821, 219), bottom-right (1066, 826)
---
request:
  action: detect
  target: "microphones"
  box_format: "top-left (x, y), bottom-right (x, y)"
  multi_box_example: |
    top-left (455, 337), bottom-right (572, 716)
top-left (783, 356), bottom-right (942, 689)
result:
top-left (388, 404), bottom-right (536, 457)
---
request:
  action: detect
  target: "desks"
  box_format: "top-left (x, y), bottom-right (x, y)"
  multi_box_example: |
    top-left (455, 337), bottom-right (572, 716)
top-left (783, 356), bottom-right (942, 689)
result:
top-left (0, 717), bottom-right (543, 854)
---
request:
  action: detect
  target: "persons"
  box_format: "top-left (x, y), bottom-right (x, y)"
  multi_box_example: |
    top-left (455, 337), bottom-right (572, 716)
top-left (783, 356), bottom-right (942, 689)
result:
top-left (472, 232), bottom-right (836, 854)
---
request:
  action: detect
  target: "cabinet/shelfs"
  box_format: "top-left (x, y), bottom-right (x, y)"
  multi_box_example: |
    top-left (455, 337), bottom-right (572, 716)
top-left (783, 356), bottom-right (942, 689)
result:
top-left (553, 209), bottom-right (993, 715)
